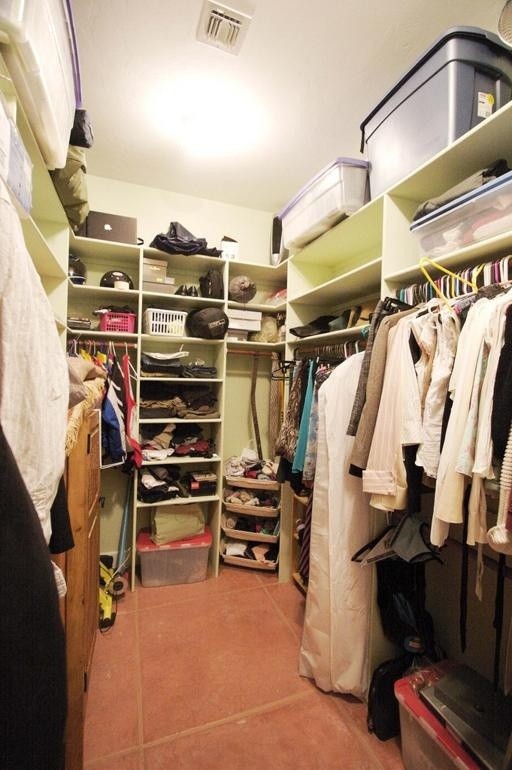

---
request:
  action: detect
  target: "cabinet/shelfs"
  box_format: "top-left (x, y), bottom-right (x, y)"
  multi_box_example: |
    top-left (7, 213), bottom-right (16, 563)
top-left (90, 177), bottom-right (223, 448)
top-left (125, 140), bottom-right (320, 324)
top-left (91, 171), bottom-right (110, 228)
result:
top-left (0, 48), bottom-right (139, 337)
top-left (66, 407), bottom-right (102, 770)
top-left (219, 474), bottom-right (281, 571)
top-left (226, 97), bottom-right (512, 600)
top-left (131, 247), bottom-right (228, 590)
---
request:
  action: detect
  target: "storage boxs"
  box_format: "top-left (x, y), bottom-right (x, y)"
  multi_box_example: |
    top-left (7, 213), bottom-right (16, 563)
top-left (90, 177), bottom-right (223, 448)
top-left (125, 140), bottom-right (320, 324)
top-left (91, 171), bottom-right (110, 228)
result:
top-left (143, 255), bottom-right (175, 294)
top-left (136, 524), bottom-right (211, 586)
top-left (359, 24), bottom-right (512, 200)
top-left (227, 309), bottom-right (262, 342)
top-left (390, 659), bottom-right (512, 770)
top-left (87, 210), bottom-right (137, 245)
top-left (410, 170), bottom-right (512, 257)
top-left (278, 157), bottom-right (368, 250)
top-left (0, 1), bottom-right (82, 171)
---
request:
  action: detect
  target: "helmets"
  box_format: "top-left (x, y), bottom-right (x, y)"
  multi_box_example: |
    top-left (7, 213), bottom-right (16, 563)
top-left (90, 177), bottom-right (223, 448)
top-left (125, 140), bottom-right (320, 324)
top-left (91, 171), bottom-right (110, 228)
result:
top-left (69, 254), bottom-right (87, 286)
top-left (101, 270), bottom-right (135, 289)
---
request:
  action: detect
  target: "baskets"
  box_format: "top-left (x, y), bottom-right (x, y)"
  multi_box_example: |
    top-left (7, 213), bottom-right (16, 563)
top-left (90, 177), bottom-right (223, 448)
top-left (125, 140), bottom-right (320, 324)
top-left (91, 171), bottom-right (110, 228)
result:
top-left (99, 308), bottom-right (188, 336)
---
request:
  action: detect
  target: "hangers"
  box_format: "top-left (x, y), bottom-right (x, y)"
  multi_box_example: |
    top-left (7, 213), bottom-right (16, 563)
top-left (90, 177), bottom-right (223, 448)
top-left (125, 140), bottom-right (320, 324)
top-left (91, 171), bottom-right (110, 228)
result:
top-left (271, 338), bottom-right (367, 384)
top-left (388, 254), bottom-right (512, 331)
top-left (66, 339), bottom-right (137, 392)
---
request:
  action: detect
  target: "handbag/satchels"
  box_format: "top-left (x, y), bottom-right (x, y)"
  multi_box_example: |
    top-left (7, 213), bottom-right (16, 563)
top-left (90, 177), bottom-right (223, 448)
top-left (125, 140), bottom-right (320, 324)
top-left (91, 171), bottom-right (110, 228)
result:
top-left (366, 655), bottom-right (412, 741)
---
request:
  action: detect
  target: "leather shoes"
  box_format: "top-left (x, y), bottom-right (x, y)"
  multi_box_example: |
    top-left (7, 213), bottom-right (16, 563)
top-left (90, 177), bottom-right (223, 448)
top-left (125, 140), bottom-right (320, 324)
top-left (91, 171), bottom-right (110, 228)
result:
top-left (176, 284), bottom-right (197, 297)
